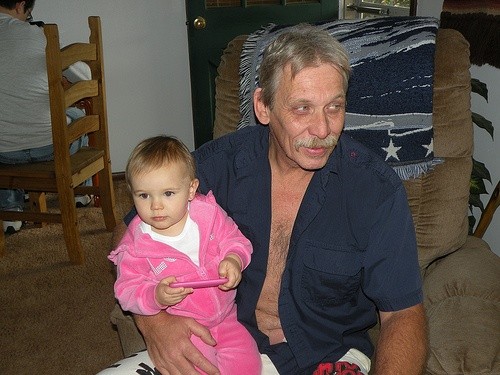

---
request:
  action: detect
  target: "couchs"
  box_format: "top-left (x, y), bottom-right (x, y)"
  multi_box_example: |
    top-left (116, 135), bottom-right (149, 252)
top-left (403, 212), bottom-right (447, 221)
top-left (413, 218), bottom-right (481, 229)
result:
top-left (108, 29), bottom-right (500, 375)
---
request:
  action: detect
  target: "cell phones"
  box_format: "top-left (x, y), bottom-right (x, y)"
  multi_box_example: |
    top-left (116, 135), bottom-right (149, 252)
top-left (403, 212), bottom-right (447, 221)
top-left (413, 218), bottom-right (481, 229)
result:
top-left (170, 279), bottom-right (228, 288)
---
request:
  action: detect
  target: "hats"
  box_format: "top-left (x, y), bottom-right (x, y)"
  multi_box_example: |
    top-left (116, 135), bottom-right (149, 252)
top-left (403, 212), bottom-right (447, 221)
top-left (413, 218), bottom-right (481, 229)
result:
top-left (63, 60), bottom-right (92, 84)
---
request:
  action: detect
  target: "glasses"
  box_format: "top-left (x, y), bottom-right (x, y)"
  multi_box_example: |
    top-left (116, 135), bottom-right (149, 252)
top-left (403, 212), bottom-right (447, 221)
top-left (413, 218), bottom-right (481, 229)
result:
top-left (26, 7), bottom-right (33, 22)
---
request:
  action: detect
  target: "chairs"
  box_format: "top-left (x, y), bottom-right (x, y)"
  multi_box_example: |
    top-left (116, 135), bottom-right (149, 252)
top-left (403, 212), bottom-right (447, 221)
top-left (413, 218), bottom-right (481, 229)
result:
top-left (0, 16), bottom-right (122, 266)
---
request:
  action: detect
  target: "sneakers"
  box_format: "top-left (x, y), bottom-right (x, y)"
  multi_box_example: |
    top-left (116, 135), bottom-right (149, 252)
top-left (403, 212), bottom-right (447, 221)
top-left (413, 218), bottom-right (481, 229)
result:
top-left (75, 194), bottom-right (94, 207)
top-left (2, 208), bottom-right (22, 232)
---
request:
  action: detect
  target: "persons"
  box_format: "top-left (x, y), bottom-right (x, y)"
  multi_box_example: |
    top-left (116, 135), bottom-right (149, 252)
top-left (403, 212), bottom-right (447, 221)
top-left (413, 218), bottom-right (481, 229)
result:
top-left (93, 25), bottom-right (428, 375)
top-left (0, 0), bottom-right (95, 233)
top-left (107, 136), bottom-right (261, 375)
